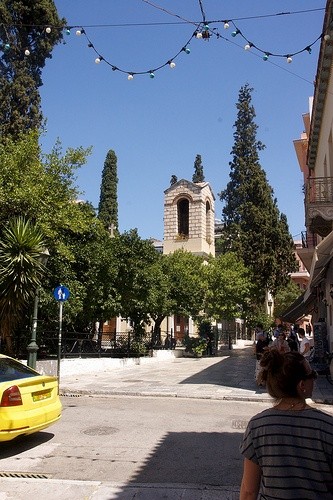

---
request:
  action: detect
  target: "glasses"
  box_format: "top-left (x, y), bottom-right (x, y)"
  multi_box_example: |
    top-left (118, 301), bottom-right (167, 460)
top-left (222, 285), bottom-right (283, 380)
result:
top-left (279, 334), bottom-right (283, 336)
top-left (302, 369), bottom-right (319, 383)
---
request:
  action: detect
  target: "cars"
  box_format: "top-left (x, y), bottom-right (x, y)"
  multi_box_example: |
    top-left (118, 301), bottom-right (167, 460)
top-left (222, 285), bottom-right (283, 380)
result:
top-left (0, 354), bottom-right (63, 443)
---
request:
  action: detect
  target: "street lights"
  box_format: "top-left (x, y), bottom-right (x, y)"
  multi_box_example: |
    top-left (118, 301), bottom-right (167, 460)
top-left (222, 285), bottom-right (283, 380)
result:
top-left (268, 297), bottom-right (272, 317)
top-left (27, 247), bottom-right (50, 370)
